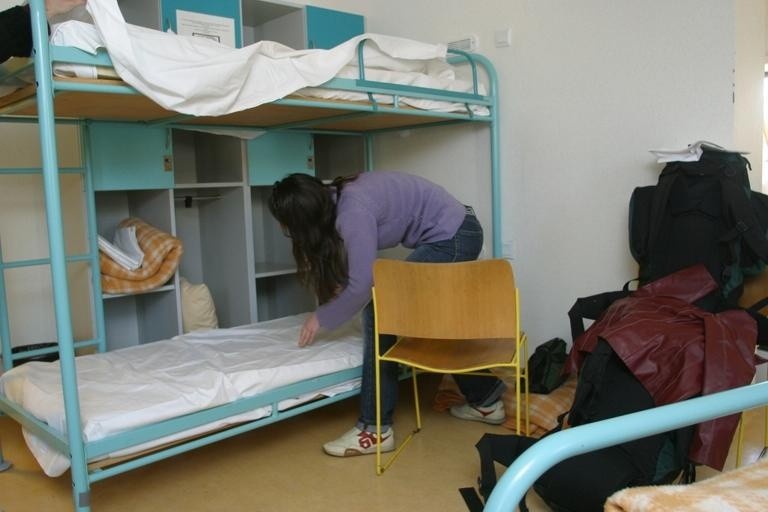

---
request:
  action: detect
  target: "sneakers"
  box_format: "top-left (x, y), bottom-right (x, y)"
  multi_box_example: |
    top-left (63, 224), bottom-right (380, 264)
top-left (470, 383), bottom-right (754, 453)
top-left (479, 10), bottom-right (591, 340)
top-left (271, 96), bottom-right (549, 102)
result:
top-left (450, 397), bottom-right (506, 425)
top-left (323, 426), bottom-right (396, 457)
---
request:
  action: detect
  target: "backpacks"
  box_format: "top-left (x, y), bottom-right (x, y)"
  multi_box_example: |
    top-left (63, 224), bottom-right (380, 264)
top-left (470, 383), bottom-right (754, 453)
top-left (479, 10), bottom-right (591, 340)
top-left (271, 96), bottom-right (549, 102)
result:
top-left (476, 339), bottom-right (701, 512)
top-left (629, 145), bottom-right (768, 313)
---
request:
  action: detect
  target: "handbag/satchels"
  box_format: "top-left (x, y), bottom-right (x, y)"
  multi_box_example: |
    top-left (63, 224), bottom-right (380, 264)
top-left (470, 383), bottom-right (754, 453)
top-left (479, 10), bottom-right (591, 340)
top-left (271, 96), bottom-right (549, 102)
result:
top-left (513, 336), bottom-right (574, 398)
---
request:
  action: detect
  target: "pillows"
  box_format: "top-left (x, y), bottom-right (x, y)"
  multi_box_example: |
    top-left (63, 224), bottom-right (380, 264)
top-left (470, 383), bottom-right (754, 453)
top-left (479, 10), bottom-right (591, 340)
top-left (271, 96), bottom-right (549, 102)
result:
top-left (182, 278), bottom-right (220, 333)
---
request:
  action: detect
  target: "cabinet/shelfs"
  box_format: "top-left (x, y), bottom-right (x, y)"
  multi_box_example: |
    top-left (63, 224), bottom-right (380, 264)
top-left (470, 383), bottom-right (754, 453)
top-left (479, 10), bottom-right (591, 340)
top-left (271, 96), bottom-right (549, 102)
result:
top-left (48, 1), bottom-right (378, 355)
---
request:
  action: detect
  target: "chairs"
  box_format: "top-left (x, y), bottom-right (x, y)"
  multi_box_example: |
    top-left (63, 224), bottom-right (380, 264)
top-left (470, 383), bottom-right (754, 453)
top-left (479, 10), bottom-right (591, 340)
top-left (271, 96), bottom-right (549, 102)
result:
top-left (366, 257), bottom-right (527, 476)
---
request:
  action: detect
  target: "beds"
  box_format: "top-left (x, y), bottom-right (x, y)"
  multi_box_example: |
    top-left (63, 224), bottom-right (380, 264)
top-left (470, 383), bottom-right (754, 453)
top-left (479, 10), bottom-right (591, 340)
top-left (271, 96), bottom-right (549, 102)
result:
top-left (1, 0), bottom-right (504, 512)
top-left (482, 381), bottom-right (768, 512)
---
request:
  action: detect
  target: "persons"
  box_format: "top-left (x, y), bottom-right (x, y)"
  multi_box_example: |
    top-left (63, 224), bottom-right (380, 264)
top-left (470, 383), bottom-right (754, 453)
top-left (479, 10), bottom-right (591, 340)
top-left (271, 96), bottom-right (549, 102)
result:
top-left (260, 168), bottom-right (507, 458)
